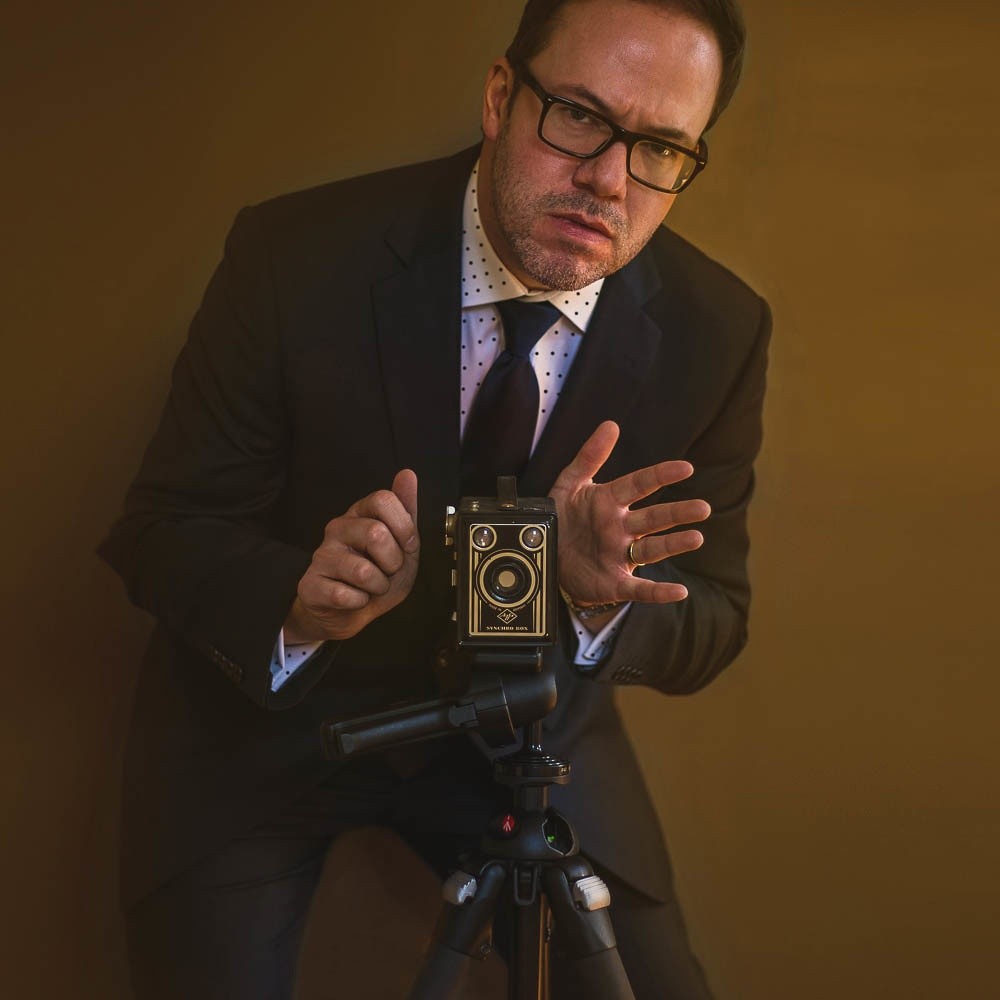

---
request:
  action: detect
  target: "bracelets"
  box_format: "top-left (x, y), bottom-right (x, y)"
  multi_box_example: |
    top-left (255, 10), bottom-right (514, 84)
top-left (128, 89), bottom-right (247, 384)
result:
top-left (558, 583), bottom-right (630, 619)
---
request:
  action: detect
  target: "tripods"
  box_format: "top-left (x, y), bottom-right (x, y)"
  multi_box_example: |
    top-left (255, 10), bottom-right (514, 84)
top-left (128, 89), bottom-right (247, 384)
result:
top-left (407, 741), bottom-right (639, 1000)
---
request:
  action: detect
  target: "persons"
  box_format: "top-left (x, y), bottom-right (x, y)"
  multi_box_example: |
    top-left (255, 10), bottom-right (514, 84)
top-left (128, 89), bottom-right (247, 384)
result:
top-left (115, 0), bottom-right (770, 1000)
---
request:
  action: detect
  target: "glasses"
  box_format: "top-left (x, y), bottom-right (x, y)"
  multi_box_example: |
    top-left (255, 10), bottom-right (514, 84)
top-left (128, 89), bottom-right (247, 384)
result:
top-left (505, 57), bottom-right (707, 196)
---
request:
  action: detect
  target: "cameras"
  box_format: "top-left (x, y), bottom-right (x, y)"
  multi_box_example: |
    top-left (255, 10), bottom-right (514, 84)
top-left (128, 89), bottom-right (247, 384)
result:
top-left (436, 491), bottom-right (555, 654)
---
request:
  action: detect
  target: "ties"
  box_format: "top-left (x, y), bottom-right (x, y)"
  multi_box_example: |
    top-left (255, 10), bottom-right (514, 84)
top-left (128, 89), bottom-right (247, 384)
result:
top-left (459, 300), bottom-right (566, 503)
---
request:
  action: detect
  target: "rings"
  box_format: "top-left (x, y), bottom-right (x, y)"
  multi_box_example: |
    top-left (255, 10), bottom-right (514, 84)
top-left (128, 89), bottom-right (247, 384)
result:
top-left (629, 538), bottom-right (648, 569)
